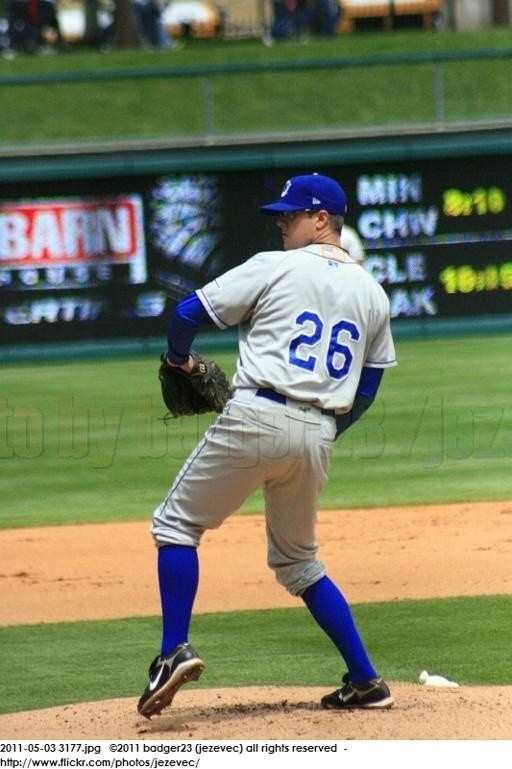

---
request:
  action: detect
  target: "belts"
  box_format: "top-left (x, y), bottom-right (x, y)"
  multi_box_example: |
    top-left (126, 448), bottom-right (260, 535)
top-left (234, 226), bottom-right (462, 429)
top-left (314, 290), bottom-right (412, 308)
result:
top-left (255, 386), bottom-right (336, 420)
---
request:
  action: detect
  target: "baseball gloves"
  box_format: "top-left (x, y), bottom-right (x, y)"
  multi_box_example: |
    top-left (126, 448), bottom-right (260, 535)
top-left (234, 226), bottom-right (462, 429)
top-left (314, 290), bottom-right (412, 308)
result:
top-left (159, 350), bottom-right (229, 419)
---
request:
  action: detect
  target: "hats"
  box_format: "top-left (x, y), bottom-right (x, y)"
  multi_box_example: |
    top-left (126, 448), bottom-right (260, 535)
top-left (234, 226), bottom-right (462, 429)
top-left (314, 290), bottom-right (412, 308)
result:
top-left (258, 172), bottom-right (347, 217)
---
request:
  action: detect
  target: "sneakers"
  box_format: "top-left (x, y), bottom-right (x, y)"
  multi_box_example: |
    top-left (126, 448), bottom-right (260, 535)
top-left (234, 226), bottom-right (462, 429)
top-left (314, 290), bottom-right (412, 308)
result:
top-left (137, 638), bottom-right (206, 720)
top-left (320, 675), bottom-right (395, 711)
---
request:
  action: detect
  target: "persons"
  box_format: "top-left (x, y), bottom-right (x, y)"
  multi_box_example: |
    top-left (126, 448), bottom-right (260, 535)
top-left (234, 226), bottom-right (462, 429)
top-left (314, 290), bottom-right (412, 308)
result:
top-left (136, 170), bottom-right (402, 721)
